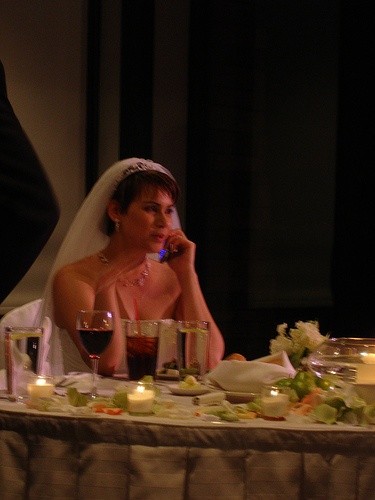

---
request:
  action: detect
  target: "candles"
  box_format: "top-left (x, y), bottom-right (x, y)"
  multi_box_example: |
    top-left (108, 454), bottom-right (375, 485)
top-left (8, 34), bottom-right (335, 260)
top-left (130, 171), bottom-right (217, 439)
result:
top-left (126, 384), bottom-right (155, 413)
top-left (264, 390), bottom-right (289, 417)
top-left (27, 375), bottom-right (54, 401)
top-left (355, 353), bottom-right (375, 384)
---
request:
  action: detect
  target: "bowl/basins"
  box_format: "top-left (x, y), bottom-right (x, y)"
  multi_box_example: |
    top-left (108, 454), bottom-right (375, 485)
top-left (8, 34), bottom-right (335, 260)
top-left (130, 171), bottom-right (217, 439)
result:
top-left (328, 336), bottom-right (375, 356)
top-left (308, 359), bottom-right (375, 406)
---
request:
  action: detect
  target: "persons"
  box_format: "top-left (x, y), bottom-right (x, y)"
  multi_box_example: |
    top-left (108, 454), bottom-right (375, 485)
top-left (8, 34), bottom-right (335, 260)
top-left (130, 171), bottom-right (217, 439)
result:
top-left (35, 157), bottom-right (224, 377)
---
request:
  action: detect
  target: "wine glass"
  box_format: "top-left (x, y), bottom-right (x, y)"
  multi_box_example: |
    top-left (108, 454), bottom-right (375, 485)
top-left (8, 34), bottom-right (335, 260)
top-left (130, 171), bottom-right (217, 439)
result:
top-left (75, 309), bottom-right (114, 401)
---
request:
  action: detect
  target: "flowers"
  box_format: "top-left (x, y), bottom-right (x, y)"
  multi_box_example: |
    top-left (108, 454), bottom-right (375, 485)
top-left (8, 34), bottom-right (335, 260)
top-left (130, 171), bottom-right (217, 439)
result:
top-left (270, 320), bottom-right (327, 361)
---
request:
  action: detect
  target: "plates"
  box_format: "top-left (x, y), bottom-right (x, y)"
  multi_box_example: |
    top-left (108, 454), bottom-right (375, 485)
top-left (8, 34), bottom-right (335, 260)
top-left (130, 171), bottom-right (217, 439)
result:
top-left (167, 383), bottom-right (210, 396)
top-left (211, 388), bottom-right (288, 405)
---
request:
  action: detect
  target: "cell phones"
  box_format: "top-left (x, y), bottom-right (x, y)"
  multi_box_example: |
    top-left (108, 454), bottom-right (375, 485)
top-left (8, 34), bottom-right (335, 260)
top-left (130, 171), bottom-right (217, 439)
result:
top-left (160, 248), bottom-right (170, 264)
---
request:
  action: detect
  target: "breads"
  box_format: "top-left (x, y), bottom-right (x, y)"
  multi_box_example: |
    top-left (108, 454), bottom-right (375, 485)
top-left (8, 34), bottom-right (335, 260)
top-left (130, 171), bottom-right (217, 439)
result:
top-left (224, 353), bottom-right (246, 361)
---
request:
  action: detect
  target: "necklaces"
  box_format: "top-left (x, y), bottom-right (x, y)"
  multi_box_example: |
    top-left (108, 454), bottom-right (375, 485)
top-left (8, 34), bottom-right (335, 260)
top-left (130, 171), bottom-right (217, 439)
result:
top-left (97, 252), bottom-right (151, 287)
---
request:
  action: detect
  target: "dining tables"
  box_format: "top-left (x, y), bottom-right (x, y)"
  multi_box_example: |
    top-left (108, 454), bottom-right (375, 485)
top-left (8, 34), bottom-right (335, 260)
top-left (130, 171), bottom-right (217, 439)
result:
top-left (0, 373), bottom-right (375, 500)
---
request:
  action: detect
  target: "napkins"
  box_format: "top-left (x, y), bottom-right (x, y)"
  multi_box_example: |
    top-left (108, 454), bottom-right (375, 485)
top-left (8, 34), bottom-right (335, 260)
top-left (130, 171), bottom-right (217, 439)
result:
top-left (203, 350), bottom-right (296, 393)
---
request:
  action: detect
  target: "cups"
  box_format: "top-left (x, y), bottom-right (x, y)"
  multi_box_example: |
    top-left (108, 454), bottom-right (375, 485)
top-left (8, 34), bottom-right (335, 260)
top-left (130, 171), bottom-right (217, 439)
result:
top-left (261, 385), bottom-right (290, 421)
top-left (5, 325), bottom-right (45, 404)
top-left (126, 381), bottom-right (157, 417)
top-left (25, 377), bottom-right (57, 401)
top-left (124, 320), bottom-right (160, 383)
top-left (177, 320), bottom-right (209, 383)
